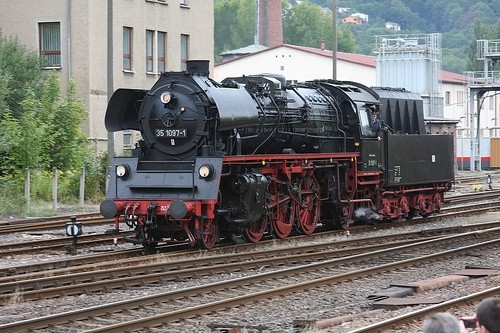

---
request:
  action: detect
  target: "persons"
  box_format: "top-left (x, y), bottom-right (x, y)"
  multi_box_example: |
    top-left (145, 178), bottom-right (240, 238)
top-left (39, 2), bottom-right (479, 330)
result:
top-left (423, 297), bottom-right (500, 333)
top-left (371, 111), bottom-right (381, 141)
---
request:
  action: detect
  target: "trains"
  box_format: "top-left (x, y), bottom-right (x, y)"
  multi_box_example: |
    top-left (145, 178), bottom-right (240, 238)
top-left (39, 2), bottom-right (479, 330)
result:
top-left (100, 60), bottom-right (455, 249)
